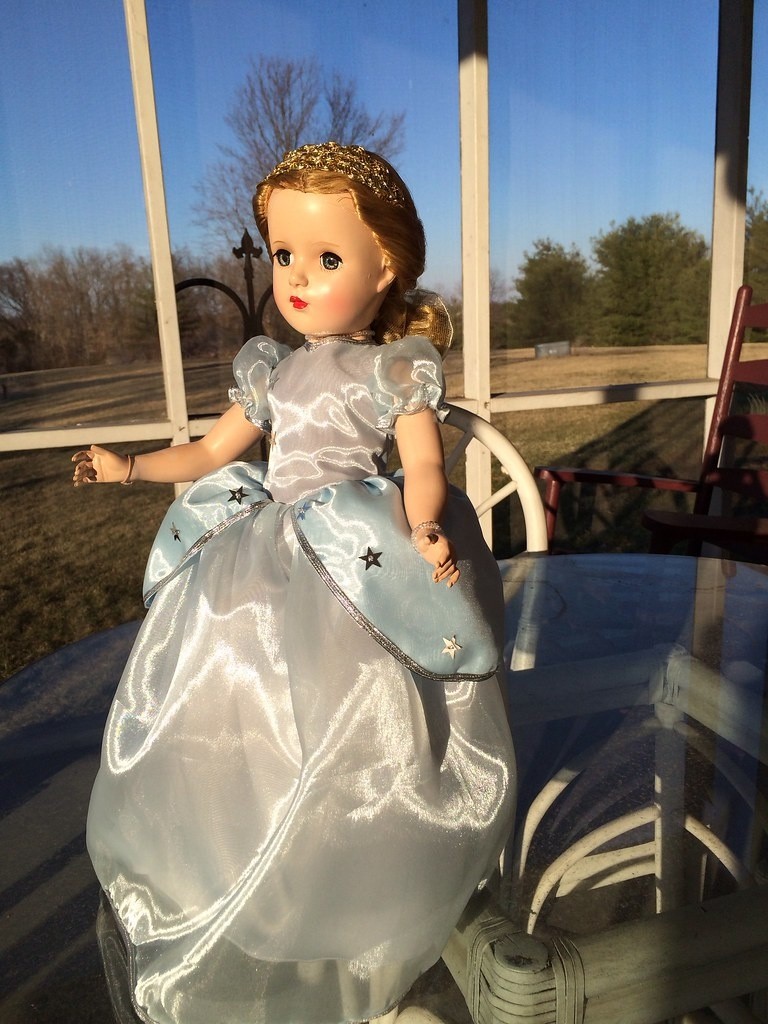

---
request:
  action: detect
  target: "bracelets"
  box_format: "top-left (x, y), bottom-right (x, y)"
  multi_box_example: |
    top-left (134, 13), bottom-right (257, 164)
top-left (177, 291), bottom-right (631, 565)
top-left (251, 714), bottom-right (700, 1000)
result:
top-left (121, 454), bottom-right (133, 485)
top-left (411, 521), bottom-right (441, 554)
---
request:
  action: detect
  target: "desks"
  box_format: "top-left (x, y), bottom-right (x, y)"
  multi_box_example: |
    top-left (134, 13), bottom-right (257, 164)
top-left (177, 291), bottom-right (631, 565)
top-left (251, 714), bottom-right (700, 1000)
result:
top-left (0, 554), bottom-right (768, 1024)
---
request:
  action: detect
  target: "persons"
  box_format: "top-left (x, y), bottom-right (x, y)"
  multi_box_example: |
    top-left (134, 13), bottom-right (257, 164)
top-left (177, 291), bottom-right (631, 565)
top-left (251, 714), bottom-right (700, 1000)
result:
top-left (71, 143), bottom-right (516, 1024)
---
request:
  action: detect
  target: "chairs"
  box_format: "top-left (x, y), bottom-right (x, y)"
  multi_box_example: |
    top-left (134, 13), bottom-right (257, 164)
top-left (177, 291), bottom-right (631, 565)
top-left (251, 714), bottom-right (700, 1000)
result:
top-left (533, 283), bottom-right (767, 566)
top-left (437, 400), bottom-right (549, 673)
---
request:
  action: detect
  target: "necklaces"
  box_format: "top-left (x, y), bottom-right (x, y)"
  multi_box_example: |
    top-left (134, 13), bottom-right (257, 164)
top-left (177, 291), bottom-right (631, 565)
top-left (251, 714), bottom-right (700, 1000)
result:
top-left (305, 330), bottom-right (375, 339)
